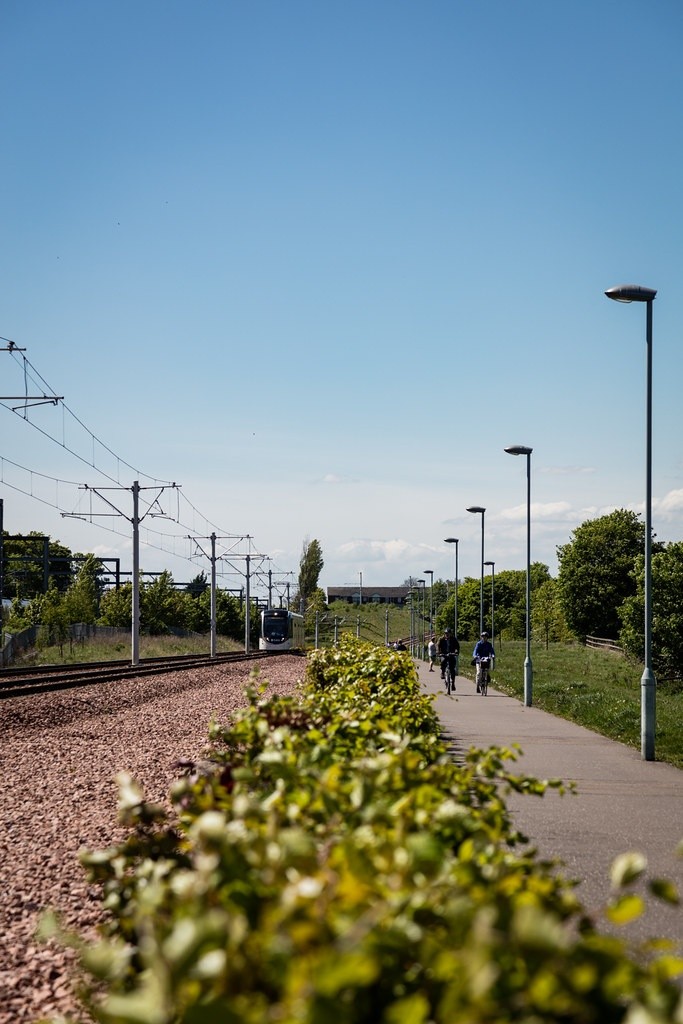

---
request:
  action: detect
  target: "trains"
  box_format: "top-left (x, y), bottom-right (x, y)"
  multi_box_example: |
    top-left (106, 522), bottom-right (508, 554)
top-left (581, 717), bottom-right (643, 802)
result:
top-left (261, 609), bottom-right (307, 652)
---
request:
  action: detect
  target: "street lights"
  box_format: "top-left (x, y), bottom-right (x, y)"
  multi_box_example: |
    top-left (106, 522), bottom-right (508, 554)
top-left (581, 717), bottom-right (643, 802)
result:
top-left (604, 286), bottom-right (658, 762)
top-left (504, 445), bottom-right (536, 707)
top-left (405, 591), bottom-right (416, 657)
top-left (423, 569), bottom-right (433, 638)
top-left (444, 537), bottom-right (458, 640)
top-left (412, 586), bottom-right (420, 659)
top-left (483, 560), bottom-right (497, 653)
top-left (416, 580), bottom-right (427, 661)
top-left (466, 505), bottom-right (485, 640)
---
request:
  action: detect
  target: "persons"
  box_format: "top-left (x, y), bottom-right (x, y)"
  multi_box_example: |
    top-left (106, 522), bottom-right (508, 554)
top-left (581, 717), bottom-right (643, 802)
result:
top-left (473, 632), bottom-right (495, 693)
top-left (437, 628), bottom-right (460, 691)
top-left (393, 641), bottom-right (398, 648)
top-left (428, 637), bottom-right (436, 672)
top-left (397, 641), bottom-right (407, 651)
top-left (386, 642), bottom-right (391, 647)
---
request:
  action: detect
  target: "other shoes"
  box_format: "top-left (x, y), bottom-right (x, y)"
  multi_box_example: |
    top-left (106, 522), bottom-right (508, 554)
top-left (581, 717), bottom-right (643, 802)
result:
top-left (452, 684), bottom-right (455, 690)
top-left (487, 675), bottom-right (490, 682)
top-left (477, 689), bottom-right (480, 693)
top-left (441, 674), bottom-right (445, 678)
top-left (429, 670), bottom-right (434, 672)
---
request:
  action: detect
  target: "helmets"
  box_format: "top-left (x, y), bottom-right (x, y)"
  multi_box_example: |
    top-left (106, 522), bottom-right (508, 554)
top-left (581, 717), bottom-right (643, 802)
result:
top-left (444, 628), bottom-right (451, 633)
top-left (481, 632), bottom-right (489, 637)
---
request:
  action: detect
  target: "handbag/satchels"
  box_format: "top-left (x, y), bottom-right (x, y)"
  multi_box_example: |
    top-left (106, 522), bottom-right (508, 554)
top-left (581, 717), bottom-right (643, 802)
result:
top-left (471, 659), bottom-right (476, 666)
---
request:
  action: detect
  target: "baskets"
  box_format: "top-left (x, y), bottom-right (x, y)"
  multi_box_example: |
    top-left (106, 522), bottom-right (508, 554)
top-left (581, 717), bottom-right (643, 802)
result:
top-left (480, 661), bottom-right (490, 669)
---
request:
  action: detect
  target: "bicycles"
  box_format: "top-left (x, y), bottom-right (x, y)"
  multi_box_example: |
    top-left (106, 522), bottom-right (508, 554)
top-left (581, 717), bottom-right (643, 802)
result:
top-left (437, 652), bottom-right (460, 695)
top-left (475, 655), bottom-right (495, 695)
